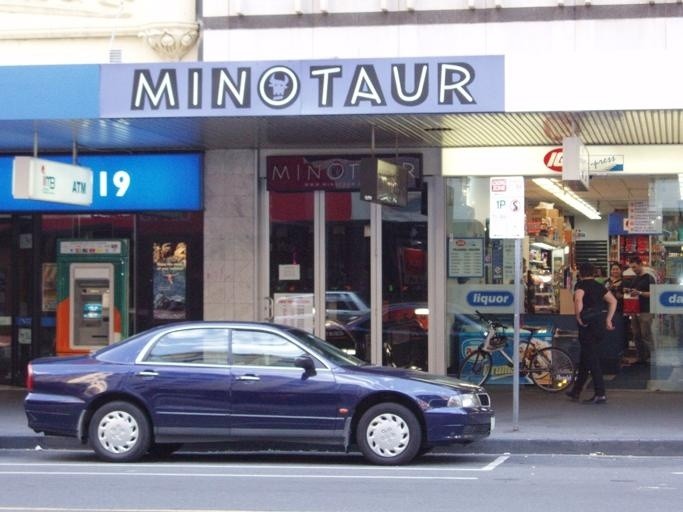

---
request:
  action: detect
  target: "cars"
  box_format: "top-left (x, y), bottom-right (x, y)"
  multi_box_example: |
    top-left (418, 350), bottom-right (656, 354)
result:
top-left (344, 300), bottom-right (581, 380)
top-left (21, 318), bottom-right (500, 468)
top-left (311, 290), bottom-right (369, 317)
top-left (322, 318), bottom-right (358, 356)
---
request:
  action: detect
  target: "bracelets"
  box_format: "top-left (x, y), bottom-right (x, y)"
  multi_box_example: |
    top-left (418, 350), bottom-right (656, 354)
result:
top-left (639, 291), bottom-right (642, 295)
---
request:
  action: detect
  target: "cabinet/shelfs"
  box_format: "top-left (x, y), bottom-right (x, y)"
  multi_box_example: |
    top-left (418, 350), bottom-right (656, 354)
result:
top-left (603, 233), bottom-right (681, 284)
top-left (529, 234), bottom-right (572, 315)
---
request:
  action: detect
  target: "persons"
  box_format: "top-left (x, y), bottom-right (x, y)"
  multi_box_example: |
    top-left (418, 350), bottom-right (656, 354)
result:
top-left (563, 262), bottom-right (618, 406)
top-left (532, 263), bottom-right (628, 341)
top-left (622, 258), bottom-right (657, 367)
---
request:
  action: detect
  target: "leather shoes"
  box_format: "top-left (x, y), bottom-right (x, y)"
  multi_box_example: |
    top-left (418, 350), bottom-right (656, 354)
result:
top-left (583, 394), bottom-right (607, 405)
top-left (564, 391), bottom-right (579, 399)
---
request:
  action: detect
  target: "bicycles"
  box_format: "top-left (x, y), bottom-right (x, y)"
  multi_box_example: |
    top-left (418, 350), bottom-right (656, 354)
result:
top-left (455, 308), bottom-right (578, 394)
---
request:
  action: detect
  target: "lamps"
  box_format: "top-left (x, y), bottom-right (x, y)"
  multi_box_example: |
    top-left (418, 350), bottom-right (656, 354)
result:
top-left (529, 177), bottom-right (602, 220)
